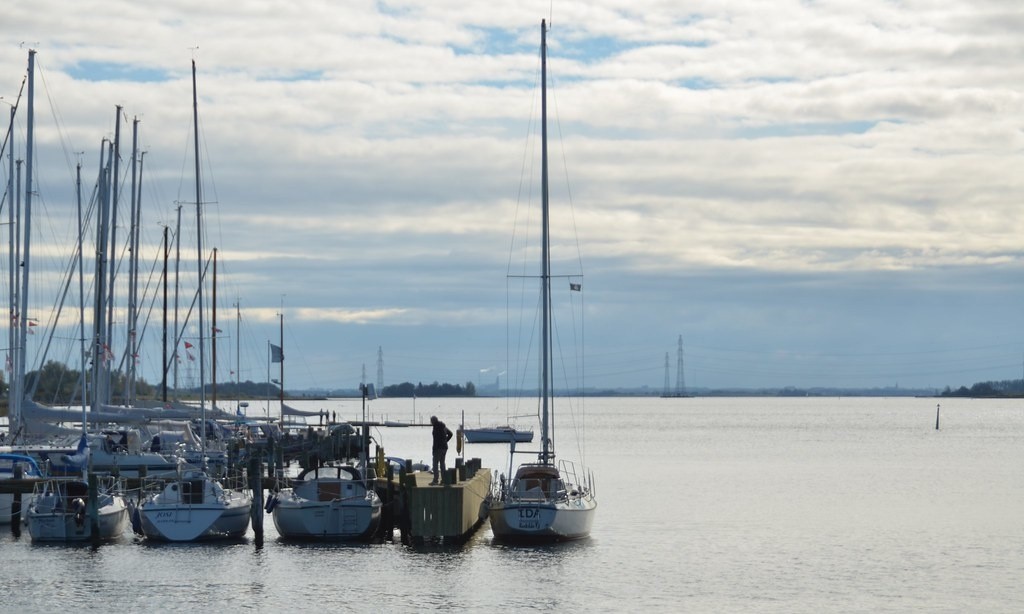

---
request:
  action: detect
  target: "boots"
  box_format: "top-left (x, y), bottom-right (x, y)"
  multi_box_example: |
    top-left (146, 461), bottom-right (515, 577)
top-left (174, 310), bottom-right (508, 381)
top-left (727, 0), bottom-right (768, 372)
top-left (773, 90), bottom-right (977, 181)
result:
top-left (428, 472), bottom-right (438, 485)
top-left (438, 473), bottom-right (445, 486)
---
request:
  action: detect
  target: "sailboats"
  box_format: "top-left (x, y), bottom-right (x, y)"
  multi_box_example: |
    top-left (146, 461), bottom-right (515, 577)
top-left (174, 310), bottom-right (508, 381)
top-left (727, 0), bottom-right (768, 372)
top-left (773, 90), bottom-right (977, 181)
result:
top-left (0, 49), bottom-right (379, 523)
top-left (489, 2), bottom-right (597, 542)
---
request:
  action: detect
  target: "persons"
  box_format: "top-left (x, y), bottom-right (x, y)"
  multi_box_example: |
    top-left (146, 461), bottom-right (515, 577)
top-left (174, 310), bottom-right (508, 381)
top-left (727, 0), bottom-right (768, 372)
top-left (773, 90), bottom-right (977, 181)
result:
top-left (428, 416), bottom-right (453, 486)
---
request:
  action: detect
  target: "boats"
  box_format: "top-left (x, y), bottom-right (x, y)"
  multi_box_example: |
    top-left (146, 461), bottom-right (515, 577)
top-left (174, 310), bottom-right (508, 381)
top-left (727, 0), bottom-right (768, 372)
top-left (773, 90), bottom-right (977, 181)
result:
top-left (353, 457), bottom-right (430, 515)
top-left (267, 465), bottom-right (384, 541)
top-left (463, 427), bottom-right (533, 442)
top-left (127, 470), bottom-right (254, 541)
top-left (385, 420), bottom-right (409, 428)
top-left (27, 478), bottom-right (125, 545)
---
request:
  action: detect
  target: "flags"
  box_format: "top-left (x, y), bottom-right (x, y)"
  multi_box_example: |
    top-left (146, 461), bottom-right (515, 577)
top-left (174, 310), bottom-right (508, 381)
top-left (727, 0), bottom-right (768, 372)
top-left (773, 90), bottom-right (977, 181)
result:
top-left (570, 284), bottom-right (581, 291)
top-left (271, 344), bottom-right (285, 363)
top-left (5, 305), bottom-right (237, 394)
